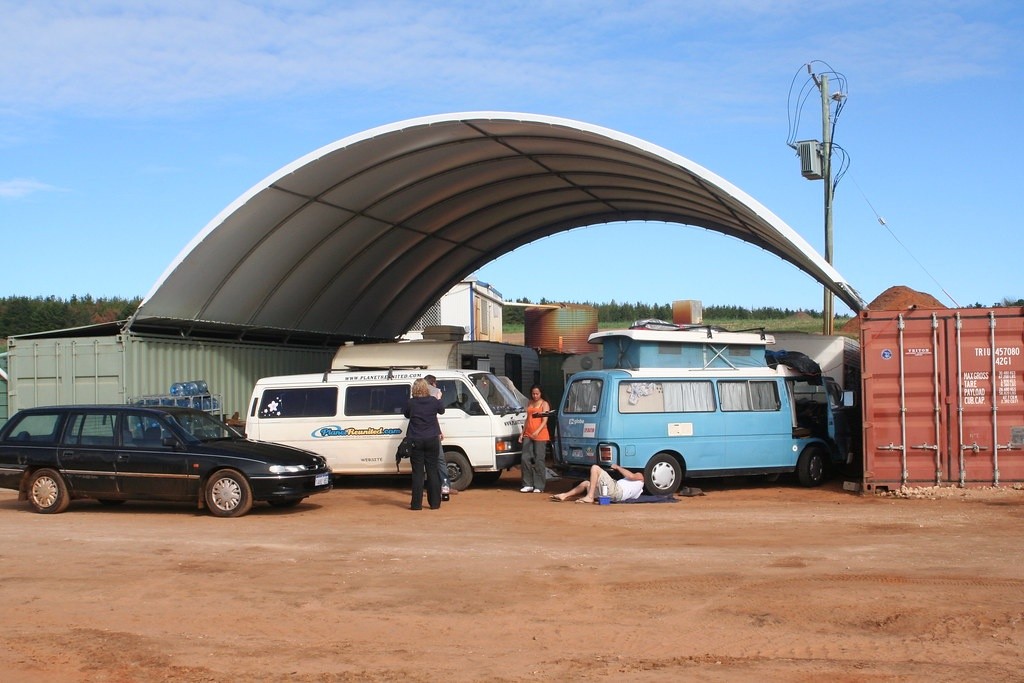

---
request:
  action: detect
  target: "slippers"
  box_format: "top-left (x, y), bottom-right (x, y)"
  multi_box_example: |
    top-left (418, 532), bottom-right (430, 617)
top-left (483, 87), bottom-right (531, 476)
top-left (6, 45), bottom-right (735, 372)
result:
top-left (575, 498), bottom-right (594, 504)
top-left (551, 494), bottom-right (560, 501)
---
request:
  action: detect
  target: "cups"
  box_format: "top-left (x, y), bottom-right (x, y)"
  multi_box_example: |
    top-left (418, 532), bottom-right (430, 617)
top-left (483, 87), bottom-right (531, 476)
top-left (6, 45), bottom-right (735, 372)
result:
top-left (602, 486), bottom-right (608, 496)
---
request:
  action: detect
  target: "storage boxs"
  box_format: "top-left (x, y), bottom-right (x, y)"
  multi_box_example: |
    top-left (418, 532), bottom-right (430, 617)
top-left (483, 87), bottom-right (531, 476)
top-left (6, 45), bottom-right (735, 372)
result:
top-left (859, 307), bottom-right (1024, 495)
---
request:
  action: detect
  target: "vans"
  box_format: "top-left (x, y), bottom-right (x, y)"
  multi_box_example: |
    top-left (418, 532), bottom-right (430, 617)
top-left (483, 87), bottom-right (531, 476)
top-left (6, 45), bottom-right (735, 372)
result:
top-left (244, 370), bottom-right (526, 491)
top-left (552, 324), bottom-right (847, 498)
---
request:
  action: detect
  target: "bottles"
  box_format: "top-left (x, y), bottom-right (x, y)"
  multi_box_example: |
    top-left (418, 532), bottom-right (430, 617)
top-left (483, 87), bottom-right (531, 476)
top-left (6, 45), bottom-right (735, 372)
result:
top-left (442, 478), bottom-right (449, 501)
top-left (132, 381), bottom-right (219, 437)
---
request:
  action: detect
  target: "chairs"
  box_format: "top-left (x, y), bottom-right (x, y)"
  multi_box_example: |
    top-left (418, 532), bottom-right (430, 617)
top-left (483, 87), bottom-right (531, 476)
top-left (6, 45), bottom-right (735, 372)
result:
top-left (122, 431), bottom-right (136, 446)
top-left (142, 427), bottom-right (161, 443)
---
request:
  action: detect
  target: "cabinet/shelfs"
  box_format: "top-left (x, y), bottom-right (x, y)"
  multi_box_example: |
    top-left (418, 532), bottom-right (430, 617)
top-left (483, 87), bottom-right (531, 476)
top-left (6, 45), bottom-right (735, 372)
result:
top-left (128, 394), bottom-right (224, 439)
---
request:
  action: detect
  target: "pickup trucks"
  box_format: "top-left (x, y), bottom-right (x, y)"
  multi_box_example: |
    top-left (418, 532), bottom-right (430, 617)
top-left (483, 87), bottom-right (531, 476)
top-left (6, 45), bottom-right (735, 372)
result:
top-left (0, 405), bottom-right (333, 519)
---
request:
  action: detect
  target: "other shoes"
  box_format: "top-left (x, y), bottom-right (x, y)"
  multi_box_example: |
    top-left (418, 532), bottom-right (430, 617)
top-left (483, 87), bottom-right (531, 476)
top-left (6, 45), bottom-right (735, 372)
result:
top-left (534, 489), bottom-right (545, 493)
top-left (422, 490), bottom-right (427, 496)
top-left (521, 487), bottom-right (533, 491)
top-left (449, 489), bottom-right (458, 494)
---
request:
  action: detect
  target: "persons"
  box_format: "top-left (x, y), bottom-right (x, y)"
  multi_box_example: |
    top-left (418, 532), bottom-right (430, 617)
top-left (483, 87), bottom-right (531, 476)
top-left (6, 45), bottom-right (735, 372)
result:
top-left (518, 384), bottom-right (551, 493)
top-left (403, 375), bottom-right (458, 510)
top-left (446, 393), bottom-right (468, 411)
top-left (549, 463), bottom-right (645, 504)
